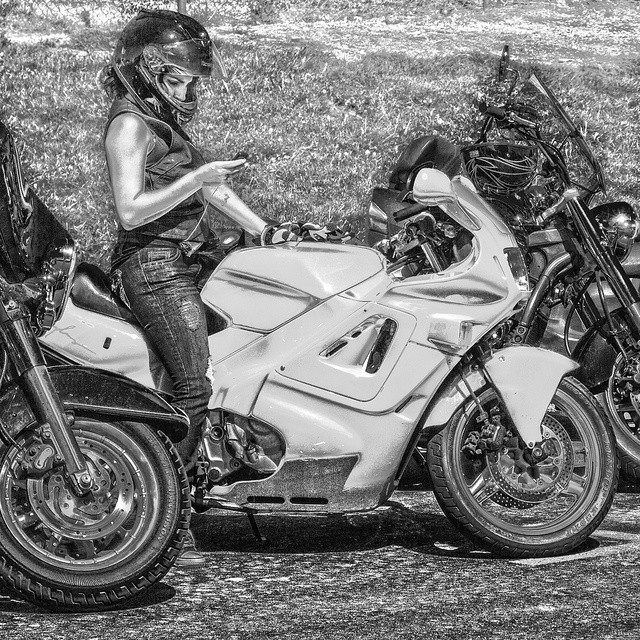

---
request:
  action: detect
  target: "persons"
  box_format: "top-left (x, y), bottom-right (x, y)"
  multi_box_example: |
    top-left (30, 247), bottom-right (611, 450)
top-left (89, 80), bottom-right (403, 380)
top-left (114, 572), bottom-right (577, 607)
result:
top-left (105, 12), bottom-right (274, 464)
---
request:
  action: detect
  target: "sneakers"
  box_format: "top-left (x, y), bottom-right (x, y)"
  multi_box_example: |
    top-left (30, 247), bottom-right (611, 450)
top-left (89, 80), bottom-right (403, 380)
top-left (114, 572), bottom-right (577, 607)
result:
top-left (176, 529), bottom-right (203, 566)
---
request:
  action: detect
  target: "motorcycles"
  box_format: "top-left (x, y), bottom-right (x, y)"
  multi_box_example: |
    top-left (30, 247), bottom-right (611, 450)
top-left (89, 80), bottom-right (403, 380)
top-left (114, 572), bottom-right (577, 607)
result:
top-left (351, 45), bottom-right (640, 484)
top-left (1, 122), bottom-right (192, 611)
top-left (41, 134), bottom-right (619, 558)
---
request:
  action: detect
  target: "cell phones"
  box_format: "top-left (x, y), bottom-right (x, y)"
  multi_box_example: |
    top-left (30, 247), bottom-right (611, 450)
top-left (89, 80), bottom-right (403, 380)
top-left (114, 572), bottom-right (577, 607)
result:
top-left (227, 151), bottom-right (249, 175)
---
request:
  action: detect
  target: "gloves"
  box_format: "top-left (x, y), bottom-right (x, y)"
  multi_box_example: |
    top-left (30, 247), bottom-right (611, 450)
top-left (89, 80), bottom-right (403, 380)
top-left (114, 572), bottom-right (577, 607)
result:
top-left (261, 222), bottom-right (354, 245)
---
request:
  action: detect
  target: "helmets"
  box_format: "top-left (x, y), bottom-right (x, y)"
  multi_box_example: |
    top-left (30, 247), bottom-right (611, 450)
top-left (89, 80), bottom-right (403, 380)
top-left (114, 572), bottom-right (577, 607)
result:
top-left (110, 8), bottom-right (212, 125)
top-left (460, 142), bottom-right (537, 193)
